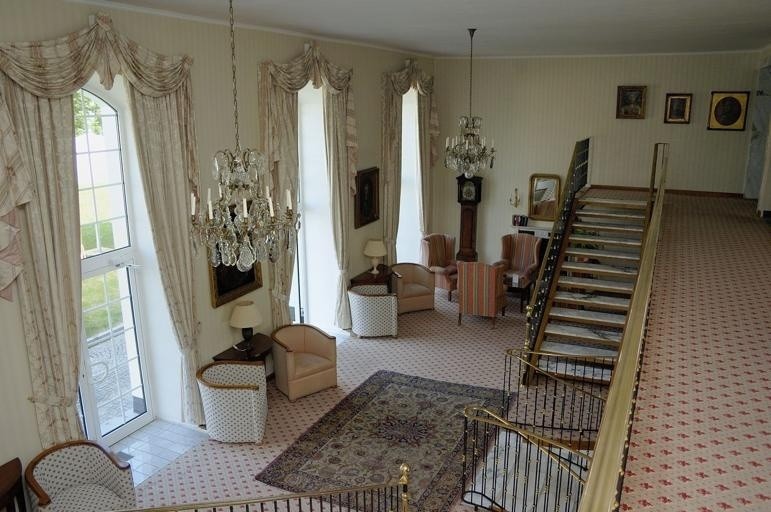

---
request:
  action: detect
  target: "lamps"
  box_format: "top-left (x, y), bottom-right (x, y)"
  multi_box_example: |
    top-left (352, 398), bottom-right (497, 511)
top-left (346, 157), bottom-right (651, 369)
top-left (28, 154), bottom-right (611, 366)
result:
top-left (441, 28), bottom-right (499, 176)
top-left (228, 300), bottom-right (264, 350)
top-left (364, 240), bottom-right (389, 274)
top-left (507, 186), bottom-right (520, 208)
top-left (186, 1), bottom-right (303, 269)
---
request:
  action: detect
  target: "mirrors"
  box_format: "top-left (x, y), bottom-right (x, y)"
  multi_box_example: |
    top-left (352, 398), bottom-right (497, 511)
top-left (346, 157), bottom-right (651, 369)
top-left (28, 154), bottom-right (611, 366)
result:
top-left (530, 173), bottom-right (562, 221)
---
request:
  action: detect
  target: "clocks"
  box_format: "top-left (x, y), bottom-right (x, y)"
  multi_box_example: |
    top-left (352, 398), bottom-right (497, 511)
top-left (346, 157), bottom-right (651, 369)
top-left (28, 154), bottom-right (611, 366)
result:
top-left (453, 172), bottom-right (484, 263)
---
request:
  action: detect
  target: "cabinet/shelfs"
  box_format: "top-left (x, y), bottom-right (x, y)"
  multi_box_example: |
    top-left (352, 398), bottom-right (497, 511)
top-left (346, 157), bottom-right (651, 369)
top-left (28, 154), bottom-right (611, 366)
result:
top-left (509, 224), bottom-right (569, 278)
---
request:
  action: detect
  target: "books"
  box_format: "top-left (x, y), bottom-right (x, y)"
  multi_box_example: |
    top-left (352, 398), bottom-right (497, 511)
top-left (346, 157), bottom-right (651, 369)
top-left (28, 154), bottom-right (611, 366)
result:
top-left (512, 214), bottom-right (528, 226)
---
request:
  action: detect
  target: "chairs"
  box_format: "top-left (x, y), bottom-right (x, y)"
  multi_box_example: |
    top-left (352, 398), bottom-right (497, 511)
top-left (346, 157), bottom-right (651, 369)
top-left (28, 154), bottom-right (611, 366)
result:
top-left (271, 323), bottom-right (340, 400)
top-left (24, 436), bottom-right (139, 510)
top-left (533, 198), bottom-right (554, 218)
top-left (421, 230), bottom-right (460, 301)
top-left (455, 258), bottom-right (507, 328)
top-left (493, 232), bottom-right (543, 317)
top-left (390, 261), bottom-right (437, 314)
top-left (347, 285), bottom-right (399, 338)
top-left (197, 358), bottom-right (269, 446)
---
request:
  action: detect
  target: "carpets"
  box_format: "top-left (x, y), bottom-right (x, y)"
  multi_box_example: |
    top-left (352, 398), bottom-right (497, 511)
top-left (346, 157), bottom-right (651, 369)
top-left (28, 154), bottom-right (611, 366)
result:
top-left (253, 366), bottom-right (519, 511)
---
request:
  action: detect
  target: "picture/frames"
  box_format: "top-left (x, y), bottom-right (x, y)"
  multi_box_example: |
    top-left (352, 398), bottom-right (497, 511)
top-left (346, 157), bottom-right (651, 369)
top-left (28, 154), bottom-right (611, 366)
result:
top-left (707, 89), bottom-right (752, 132)
top-left (205, 205), bottom-right (264, 309)
top-left (614, 84), bottom-right (649, 120)
top-left (665, 92), bottom-right (695, 124)
top-left (355, 165), bottom-right (380, 231)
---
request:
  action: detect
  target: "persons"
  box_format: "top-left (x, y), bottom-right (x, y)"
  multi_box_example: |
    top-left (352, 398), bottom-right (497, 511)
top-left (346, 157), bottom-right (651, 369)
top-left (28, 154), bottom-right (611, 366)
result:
top-left (621, 94), bottom-right (640, 115)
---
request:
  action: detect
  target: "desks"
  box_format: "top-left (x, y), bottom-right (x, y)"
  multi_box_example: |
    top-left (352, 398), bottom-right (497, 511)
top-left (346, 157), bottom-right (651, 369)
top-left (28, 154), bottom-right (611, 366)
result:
top-left (214, 331), bottom-right (276, 380)
top-left (350, 264), bottom-right (392, 288)
top-left (0, 455), bottom-right (26, 512)
